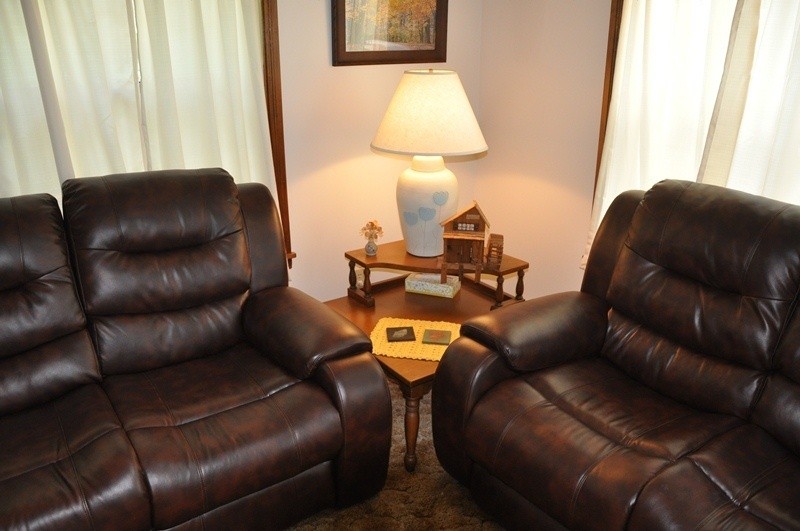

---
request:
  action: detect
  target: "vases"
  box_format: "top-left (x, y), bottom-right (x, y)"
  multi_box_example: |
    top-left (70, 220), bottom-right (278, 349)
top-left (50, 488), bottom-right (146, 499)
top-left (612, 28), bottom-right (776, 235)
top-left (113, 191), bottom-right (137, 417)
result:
top-left (365, 239), bottom-right (378, 255)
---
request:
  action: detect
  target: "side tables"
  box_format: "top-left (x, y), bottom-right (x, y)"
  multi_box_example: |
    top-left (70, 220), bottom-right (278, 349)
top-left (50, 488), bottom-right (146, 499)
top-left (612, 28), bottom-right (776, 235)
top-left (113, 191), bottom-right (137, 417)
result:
top-left (322, 238), bottom-right (530, 472)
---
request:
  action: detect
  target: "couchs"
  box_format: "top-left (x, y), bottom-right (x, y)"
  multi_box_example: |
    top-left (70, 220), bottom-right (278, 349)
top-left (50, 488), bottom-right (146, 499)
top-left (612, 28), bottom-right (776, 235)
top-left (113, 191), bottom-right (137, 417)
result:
top-left (0, 171), bottom-right (395, 530)
top-left (432, 176), bottom-right (800, 531)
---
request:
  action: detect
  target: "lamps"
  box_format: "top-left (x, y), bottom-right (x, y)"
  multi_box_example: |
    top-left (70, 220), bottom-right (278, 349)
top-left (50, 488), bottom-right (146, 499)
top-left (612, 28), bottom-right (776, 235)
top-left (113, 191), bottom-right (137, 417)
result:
top-left (371, 66), bottom-right (488, 257)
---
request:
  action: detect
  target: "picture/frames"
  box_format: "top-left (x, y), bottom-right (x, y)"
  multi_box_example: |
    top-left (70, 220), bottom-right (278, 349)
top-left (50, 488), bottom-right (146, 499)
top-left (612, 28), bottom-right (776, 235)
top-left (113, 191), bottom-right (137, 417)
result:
top-left (329, 0), bottom-right (450, 67)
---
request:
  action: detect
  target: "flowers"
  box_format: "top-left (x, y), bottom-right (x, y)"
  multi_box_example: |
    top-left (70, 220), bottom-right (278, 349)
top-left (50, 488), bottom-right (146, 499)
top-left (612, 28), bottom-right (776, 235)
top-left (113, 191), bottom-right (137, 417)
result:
top-left (361, 221), bottom-right (384, 240)
